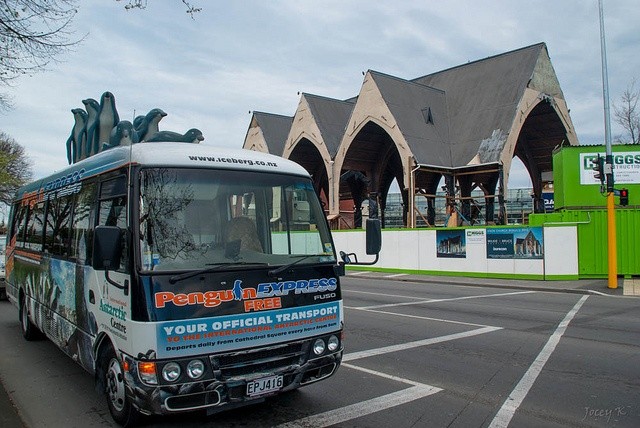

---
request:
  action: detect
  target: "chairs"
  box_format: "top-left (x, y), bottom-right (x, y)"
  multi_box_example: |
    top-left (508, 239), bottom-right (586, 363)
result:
top-left (191, 220), bottom-right (218, 249)
top-left (223, 216), bottom-right (265, 259)
top-left (457, 204), bottom-right (483, 226)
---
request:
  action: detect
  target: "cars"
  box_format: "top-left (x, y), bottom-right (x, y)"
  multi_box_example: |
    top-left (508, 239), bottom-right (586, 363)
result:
top-left (0, 235), bottom-right (10, 302)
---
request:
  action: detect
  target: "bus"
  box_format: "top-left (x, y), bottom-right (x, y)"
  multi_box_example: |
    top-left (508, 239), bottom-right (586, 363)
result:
top-left (5, 142), bottom-right (382, 426)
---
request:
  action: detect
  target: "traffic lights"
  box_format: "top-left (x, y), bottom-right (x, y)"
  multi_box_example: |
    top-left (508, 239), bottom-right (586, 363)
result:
top-left (620, 189), bottom-right (628, 206)
top-left (593, 154), bottom-right (605, 183)
